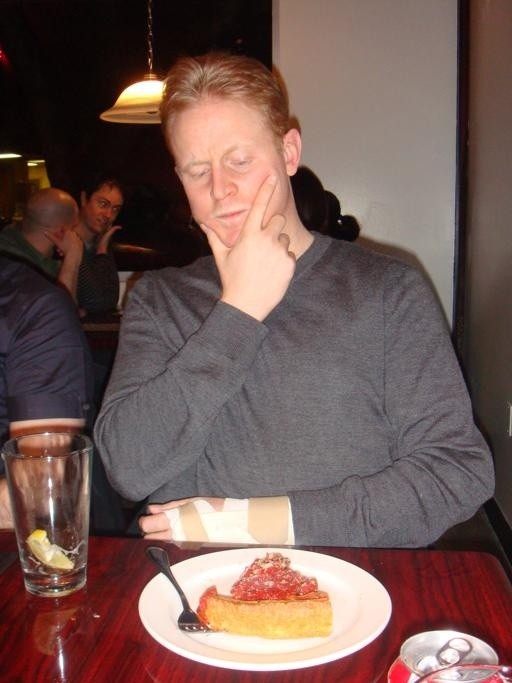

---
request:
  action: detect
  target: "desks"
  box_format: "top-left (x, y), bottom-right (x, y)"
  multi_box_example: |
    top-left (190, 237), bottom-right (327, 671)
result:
top-left (82, 310), bottom-right (121, 345)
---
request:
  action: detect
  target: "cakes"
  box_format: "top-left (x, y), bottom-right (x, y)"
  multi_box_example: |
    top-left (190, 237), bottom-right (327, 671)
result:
top-left (195, 550), bottom-right (335, 639)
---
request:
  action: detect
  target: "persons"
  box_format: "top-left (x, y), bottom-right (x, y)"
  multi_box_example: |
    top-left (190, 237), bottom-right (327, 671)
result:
top-left (92, 51), bottom-right (495, 546)
top-left (0, 187), bottom-right (83, 299)
top-left (0, 252), bottom-right (98, 529)
top-left (69, 175), bottom-right (125, 319)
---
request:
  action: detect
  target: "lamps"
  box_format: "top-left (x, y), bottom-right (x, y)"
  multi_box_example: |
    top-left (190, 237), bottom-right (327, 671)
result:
top-left (100, 0), bottom-right (170, 124)
top-left (27, 148), bottom-right (45, 163)
top-left (0, 135), bottom-right (23, 160)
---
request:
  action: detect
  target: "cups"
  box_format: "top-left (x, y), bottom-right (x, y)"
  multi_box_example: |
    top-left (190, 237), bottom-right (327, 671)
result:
top-left (407, 665), bottom-right (511, 683)
top-left (1, 429), bottom-right (96, 599)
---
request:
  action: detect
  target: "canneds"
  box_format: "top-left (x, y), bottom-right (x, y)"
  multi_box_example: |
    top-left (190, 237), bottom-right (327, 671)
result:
top-left (385, 629), bottom-right (512, 683)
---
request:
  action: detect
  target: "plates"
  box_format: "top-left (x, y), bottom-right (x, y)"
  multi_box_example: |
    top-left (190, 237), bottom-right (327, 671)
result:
top-left (137, 547), bottom-right (392, 673)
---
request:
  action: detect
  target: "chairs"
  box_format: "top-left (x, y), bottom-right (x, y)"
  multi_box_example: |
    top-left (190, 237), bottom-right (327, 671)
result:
top-left (114, 243), bottom-right (168, 271)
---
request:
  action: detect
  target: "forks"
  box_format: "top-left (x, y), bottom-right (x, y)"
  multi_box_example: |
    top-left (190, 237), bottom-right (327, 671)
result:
top-left (144, 543), bottom-right (215, 633)
